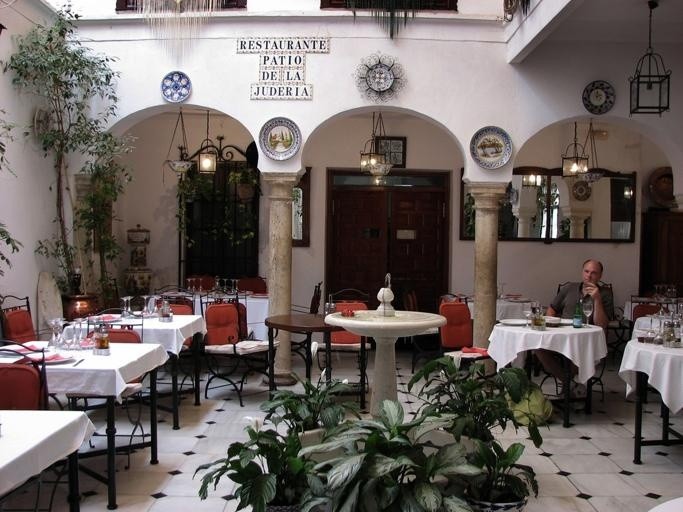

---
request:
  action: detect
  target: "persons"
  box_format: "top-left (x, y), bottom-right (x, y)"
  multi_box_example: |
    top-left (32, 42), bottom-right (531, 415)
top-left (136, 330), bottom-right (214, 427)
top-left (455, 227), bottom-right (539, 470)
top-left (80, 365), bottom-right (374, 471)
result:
top-left (544, 260), bottom-right (613, 407)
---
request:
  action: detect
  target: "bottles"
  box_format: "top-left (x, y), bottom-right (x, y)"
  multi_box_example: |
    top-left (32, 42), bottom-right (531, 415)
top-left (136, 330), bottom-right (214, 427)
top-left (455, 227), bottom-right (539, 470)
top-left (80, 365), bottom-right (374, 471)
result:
top-left (94, 326), bottom-right (109, 356)
top-left (664, 321), bottom-right (681, 347)
top-left (162, 301), bottom-right (170, 317)
top-left (325, 304), bottom-right (336, 321)
top-left (534, 312), bottom-right (544, 326)
top-left (652, 285), bottom-right (677, 297)
top-left (573, 297), bottom-right (582, 328)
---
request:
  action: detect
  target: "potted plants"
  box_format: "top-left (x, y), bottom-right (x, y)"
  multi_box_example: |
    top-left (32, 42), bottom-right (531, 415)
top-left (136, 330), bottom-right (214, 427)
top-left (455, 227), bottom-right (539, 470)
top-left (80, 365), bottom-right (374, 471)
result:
top-left (465, 440), bottom-right (539, 512)
top-left (162, 106), bottom-right (214, 248)
top-left (223, 170), bottom-right (264, 247)
top-left (0, 4), bottom-right (136, 321)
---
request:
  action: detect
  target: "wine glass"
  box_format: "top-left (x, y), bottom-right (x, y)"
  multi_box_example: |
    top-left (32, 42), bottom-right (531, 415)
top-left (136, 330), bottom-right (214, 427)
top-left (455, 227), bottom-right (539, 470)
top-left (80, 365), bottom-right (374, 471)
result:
top-left (643, 315), bottom-right (656, 343)
top-left (121, 297), bottom-right (129, 318)
top-left (654, 317), bottom-right (663, 345)
top-left (185, 277), bottom-right (240, 295)
top-left (531, 302), bottom-right (542, 317)
top-left (127, 296), bottom-right (133, 317)
top-left (583, 304), bottom-right (593, 328)
top-left (522, 303), bottom-right (532, 329)
top-left (49, 315), bottom-right (87, 353)
top-left (141, 296), bottom-right (151, 318)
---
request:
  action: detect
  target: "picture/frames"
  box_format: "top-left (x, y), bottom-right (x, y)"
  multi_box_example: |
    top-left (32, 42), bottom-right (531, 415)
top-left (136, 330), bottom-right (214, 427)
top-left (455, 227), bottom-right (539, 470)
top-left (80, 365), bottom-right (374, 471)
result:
top-left (375, 135), bottom-right (407, 167)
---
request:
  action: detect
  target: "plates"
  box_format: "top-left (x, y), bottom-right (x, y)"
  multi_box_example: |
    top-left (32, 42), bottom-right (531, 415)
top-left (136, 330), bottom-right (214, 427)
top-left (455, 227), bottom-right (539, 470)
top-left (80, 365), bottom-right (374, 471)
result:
top-left (500, 319), bottom-right (531, 325)
top-left (469, 125), bottom-right (514, 171)
top-left (87, 316), bottom-right (121, 321)
top-left (259, 116), bottom-right (302, 162)
top-left (581, 79), bottom-right (617, 116)
top-left (160, 69), bottom-right (191, 103)
top-left (561, 319), bottom-right (581, 324)
top-left (1, 342), bottom-right (73, 364)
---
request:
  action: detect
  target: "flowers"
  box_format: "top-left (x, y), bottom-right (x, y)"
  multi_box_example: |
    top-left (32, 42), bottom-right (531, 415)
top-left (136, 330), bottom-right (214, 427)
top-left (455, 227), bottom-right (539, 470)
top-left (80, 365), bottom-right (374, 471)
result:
top-left (193, 421), bottom-right (328, 512)
top-left (259, 341), bottom-right (360, 434)
top-left (407, 355), bottom-right (550, 455)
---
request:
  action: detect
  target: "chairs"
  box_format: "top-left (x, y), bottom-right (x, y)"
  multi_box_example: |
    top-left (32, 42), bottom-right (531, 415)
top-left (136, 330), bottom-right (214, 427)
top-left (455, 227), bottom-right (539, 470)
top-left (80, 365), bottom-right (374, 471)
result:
top-left (403, 291), bottom-right (438, 373)
top-left (439, 303), bottom-right (497, 376)
top-left (439, 293), bottom-right (468, 305)
top-left (291, 281), bottom-right (323, 380)
top-left (317, 288), bottom-right (372, 393)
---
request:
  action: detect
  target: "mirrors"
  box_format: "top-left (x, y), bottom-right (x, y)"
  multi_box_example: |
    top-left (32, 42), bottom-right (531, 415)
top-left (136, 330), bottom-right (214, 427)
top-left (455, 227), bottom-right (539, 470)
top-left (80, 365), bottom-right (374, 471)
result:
top-left (459, 165), bottom-right (637, 244)
top-left (291, 166), bottom-right (312, 248)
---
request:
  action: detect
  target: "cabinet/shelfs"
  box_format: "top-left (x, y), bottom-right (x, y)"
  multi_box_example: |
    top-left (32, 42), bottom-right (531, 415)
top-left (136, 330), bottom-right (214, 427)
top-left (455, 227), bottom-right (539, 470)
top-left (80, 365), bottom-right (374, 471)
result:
top-left (640, 211), bottom-right (682, 297)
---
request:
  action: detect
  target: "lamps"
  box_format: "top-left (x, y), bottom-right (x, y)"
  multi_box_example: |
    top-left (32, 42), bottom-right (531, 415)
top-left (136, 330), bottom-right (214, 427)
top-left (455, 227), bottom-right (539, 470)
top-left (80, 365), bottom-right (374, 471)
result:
top-left (360, 112), bottom-right (385, 171)
top-left (560, 122), bottom-right (590, 177)
top-left (628, 2), bottom-right (672, 118)
top-left (197, 109), bottom-right (217, 174)
